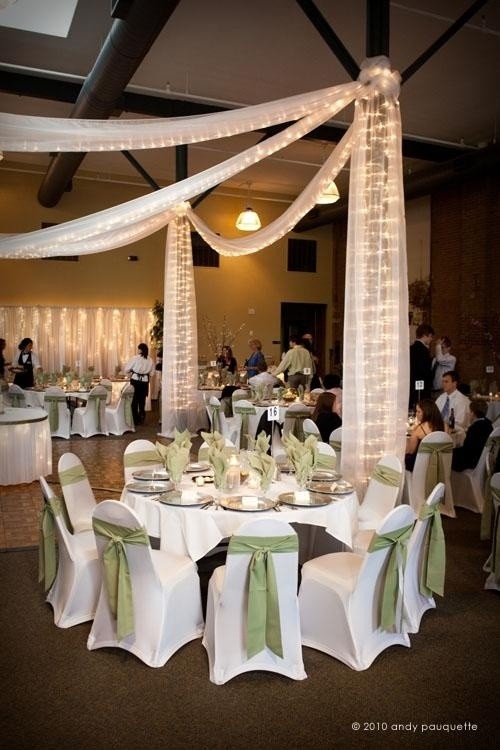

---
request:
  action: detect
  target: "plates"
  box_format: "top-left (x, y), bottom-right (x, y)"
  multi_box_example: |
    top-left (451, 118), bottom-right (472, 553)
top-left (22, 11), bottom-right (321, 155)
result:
top-left (244, 383), bottom-right (321, 408)
top-left (128, 460), bottom-right (353, 510)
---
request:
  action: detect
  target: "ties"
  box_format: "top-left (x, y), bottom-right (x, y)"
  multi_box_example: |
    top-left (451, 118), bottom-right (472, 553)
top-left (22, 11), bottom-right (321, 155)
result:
top-left (441, 399), bottom-right (449, 419)
top-left (432, 360), bottom-right (439, 382)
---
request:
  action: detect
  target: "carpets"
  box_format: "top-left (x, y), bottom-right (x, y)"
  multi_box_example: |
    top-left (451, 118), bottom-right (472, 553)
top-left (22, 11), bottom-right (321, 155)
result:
top-left (0, 505), bottom-right (500, 750)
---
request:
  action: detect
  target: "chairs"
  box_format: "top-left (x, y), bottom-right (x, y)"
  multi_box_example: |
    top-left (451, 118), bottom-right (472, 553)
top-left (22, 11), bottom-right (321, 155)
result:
top-left (0, 354), bottom-right (500, 687)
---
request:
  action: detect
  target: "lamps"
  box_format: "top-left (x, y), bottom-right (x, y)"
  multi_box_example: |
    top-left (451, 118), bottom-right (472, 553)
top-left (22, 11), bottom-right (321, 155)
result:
top-left (315, 143), bottom-right (341, 205)
top-left (236, 180), bottom-right (261, 232)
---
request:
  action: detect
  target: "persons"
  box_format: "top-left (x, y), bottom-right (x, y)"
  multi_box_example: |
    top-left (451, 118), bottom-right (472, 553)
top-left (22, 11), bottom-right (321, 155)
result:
top-left (216, 346), bottom-right (236, 375)
top-left (405, 323), bottom-right (494, 472)
top-left (323, 372), bottom-right (342, 417)
top-left (311, 392), bottom-right (342, 444)
top-left (271, 332), bottom-right (319, 389)
top-left (0, 338), bottom-right (12, 379)
top-left (12, 337), bottom-right (38, 389)
top-left (126, 343), bottom-right (153, 426)
top-left (243, 339), bottom-right (268, 378)
